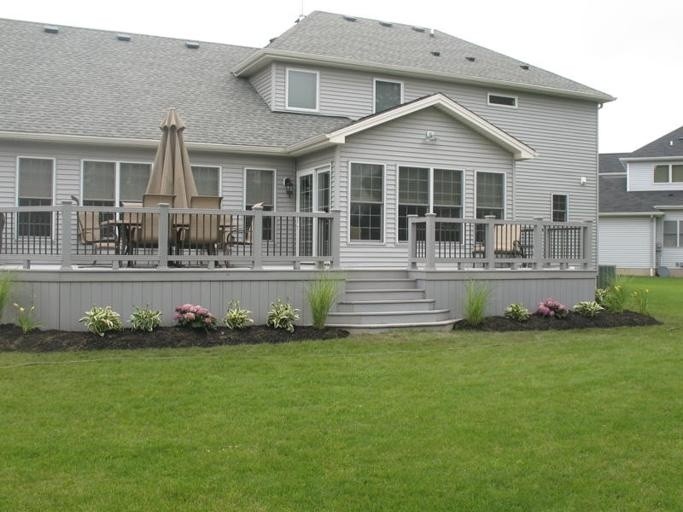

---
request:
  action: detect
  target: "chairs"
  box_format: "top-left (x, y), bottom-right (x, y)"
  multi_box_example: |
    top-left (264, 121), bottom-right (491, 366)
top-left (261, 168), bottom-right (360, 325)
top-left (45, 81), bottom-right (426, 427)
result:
top-left (70, 194), bottom-right (265, 268)
top-left (471, 224), bottom-right (523, 268)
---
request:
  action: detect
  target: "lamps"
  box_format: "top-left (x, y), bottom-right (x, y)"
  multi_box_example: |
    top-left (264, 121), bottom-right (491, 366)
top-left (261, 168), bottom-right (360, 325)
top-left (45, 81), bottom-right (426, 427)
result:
top-left (286, 181), bottom-right (293, 198)
top-left (424, 131), bottom-right (441, 143)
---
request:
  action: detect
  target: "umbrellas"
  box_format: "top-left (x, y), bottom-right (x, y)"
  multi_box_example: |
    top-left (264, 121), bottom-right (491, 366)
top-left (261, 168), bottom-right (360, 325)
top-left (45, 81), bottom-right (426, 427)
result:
top-left (145, 105), bottom-right (198, 226)
